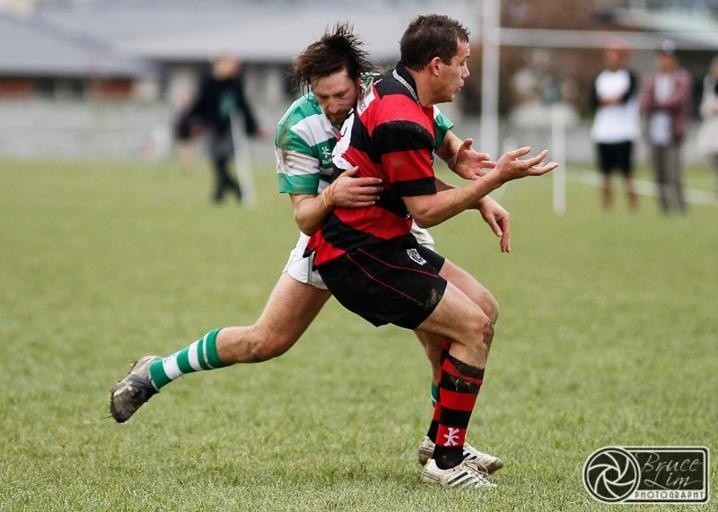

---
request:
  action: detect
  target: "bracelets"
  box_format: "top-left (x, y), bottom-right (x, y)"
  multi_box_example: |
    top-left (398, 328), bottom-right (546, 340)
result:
top-left (321, 190), bottom-right (331, 209)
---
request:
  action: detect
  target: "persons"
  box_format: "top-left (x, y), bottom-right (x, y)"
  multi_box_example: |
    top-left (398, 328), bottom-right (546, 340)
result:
top-left (589, 40), bottom-right (641, 209)
top-left (112, 20), bottom-right (506, 475)
top-left (636, 38), bottom-right (693, 214)
top-left (307, 12), bottom-right (559, 490)
top-left (172, 48), bottom-right (268, 206)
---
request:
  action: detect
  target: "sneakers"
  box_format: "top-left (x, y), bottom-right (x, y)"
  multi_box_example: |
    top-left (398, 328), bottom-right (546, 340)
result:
top-left (110, 355), bottom-right (160, 423)
top-left (418, 432), bottom-right (503, 489)
